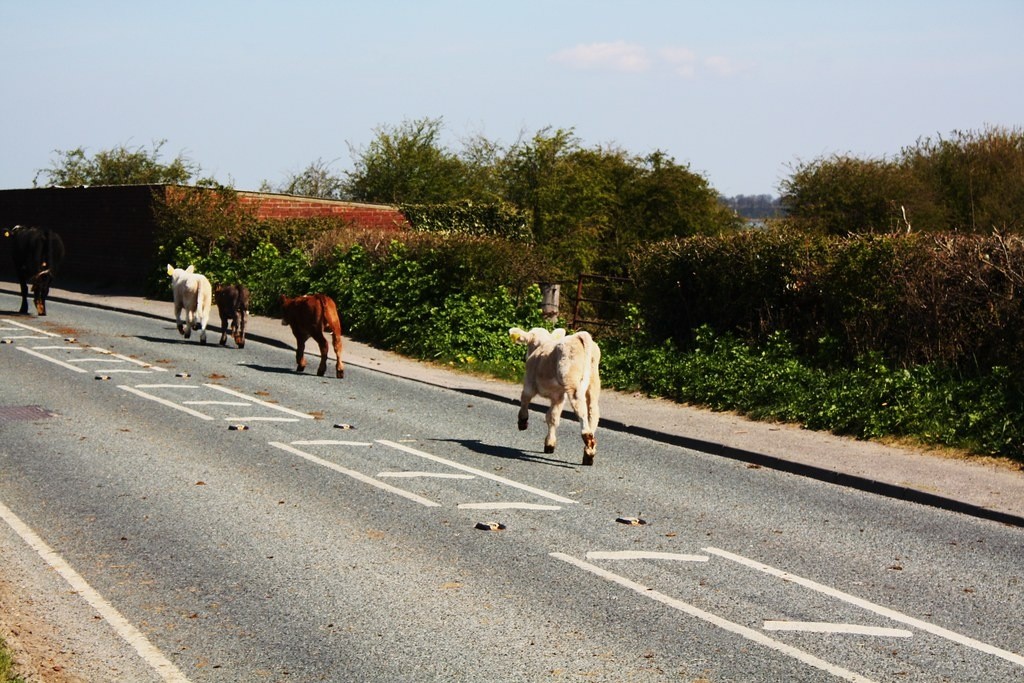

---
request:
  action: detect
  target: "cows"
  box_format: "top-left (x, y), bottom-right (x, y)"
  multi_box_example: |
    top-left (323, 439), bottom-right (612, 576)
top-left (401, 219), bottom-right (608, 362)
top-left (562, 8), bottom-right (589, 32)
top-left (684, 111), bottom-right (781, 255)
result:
top-left (281, 295), bottom-right (345, 379)
top-left (167, 263), bottom-right (213, 345)
top-left (210, 281), bottom-right (251, 348)
top-left (508, 327), bottom-right (603, 466)
top-left (0, 223), bottom-right (65, 316)
